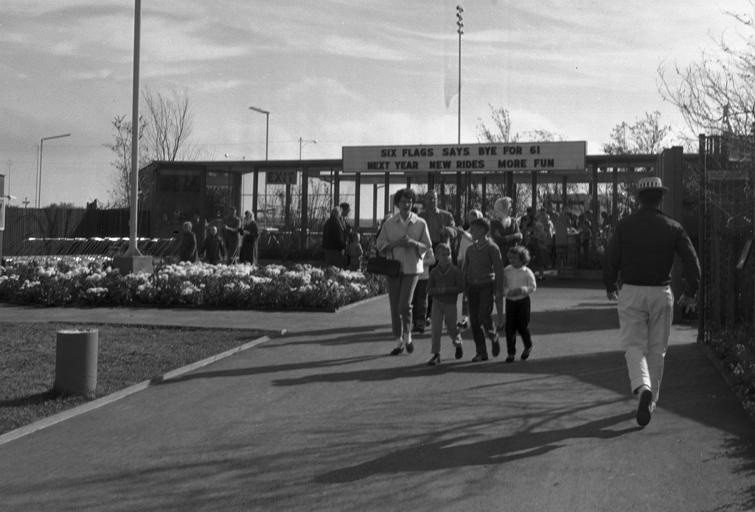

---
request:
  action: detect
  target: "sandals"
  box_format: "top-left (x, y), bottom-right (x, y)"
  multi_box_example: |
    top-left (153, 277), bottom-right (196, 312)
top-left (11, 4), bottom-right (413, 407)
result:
top-left (457, 319), bottom-right (468, 328)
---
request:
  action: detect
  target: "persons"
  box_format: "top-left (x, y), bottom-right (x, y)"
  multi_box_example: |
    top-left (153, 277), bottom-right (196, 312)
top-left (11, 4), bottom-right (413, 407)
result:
top-left (364, 184), bottom-right (550, 364)
top-left (510, 193), bottom-right (625, 270)
top-left (602, 175), bottom-right (702, 426)
top-left (166, 198), bottom-right (363, 272)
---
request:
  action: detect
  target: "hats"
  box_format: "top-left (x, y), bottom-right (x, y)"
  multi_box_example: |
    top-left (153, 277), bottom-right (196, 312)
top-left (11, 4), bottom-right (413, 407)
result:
top-left (636, 177), bottom-right (669, 193)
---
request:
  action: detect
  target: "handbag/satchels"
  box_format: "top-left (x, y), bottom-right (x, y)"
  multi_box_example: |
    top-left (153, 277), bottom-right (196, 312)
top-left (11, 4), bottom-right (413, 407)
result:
top-left (367, 257), bottom-right (401, 278)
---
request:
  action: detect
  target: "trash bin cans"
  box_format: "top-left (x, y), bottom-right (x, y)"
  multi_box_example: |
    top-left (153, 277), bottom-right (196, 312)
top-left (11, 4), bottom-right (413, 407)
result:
top-left (54, 329), bottom-right (98, 393)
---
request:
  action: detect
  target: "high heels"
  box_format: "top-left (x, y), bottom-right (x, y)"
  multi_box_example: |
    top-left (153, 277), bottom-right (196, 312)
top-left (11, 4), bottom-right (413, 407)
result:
top-left (495, 325), bottom-right (504, 332)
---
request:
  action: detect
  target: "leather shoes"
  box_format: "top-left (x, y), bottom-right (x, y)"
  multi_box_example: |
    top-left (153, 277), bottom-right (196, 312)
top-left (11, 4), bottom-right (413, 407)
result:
top-left (636, 386), bottom-right (652, 427)
top-left (506, 354), bottom-right (514, 362)
top-left (521, 345), bottom-right (533, 359)
top-left (428, 354), bottom-right (440, 365)
top-left (492, 334), bottom-right (500, 356)
top-left (406, 341), bottom-right (414, 353)
top-left (472, 352), bottom-right (488, 361)
top-left (455, 343), bottom-right (464, 359)
top-left (389, 346), bottom-right (405, 355)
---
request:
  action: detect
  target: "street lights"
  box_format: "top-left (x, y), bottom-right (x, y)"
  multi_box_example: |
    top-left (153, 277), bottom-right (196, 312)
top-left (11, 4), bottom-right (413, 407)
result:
top-left (22, 196), bottom-right (30, 208)
top-left (6, 160), bottom-right (14, 208)
top-left (225, 152), bottom-right (231, 160)
top-left (250, 104), bottom-right (271, 229)
top-left (34, 132), bottom-right (73, 235)
top-left (298, 136), bottom-right (319, 208)
top-left (4, 194), bottom-right (16, 200)
top-left (242, 156), bottom-right (246, 217)
top-left (455, 4), bottom-right (466, 231)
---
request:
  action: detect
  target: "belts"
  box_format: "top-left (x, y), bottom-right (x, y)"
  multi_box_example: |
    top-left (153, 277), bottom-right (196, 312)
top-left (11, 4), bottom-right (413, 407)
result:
top-left (622, 281), bottom-right (669, 286)
